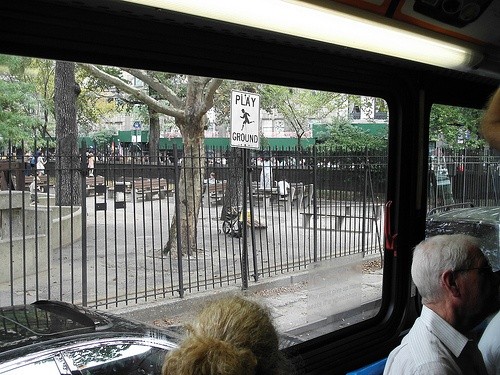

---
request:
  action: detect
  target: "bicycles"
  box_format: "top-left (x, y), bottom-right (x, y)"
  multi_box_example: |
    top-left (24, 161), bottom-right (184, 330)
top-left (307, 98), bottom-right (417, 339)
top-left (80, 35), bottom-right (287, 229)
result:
top-left (222, 206), bottom-right (243, 237)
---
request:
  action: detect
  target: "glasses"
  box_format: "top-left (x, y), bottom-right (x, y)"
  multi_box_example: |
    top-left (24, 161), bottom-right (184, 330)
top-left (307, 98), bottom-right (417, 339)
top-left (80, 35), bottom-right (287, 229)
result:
top-left (454, 264), bottom-right (493, 274)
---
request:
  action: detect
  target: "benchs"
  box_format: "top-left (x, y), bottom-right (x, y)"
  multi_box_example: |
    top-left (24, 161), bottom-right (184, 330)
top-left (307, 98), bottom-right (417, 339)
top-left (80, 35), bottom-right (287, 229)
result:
top-left (300, 212), bottom-right (340, 230)
top-left (339, 215), bottom-right (378, 231)
top-left (203, 178), bottom-right (312, 212)
top-left (345, 205), bottom-right (384, 217)
top-left (309, 205), bottom-right (345, 215)
top-left (11, 175), bottom-right (52, 192)
top-left (85, 176), bottom-right (174, 201)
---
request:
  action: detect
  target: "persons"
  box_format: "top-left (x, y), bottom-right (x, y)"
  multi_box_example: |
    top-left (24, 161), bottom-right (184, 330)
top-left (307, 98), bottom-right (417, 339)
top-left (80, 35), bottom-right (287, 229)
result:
top-left (160, 294), bottom-right (280, 375)
top-left (29, 177), bottom-right (41, 206)
top-left (382, 234), bottom-right (500, 375)
top-left (260, 153), bottom-right (274, 208)
top-left (277, 176), bottom-right (290, 198)
top-left (201, 172), bottom-right (217, 197)
top-left (29, 147), bottom-right (99, 177)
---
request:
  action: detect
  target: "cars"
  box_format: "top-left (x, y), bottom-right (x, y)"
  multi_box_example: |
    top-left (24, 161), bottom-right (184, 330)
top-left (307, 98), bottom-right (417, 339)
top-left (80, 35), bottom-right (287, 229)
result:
top-left (0, 300), bottom-right (304, 375)
top-left (424, 206), bottom-right (500, 273)
